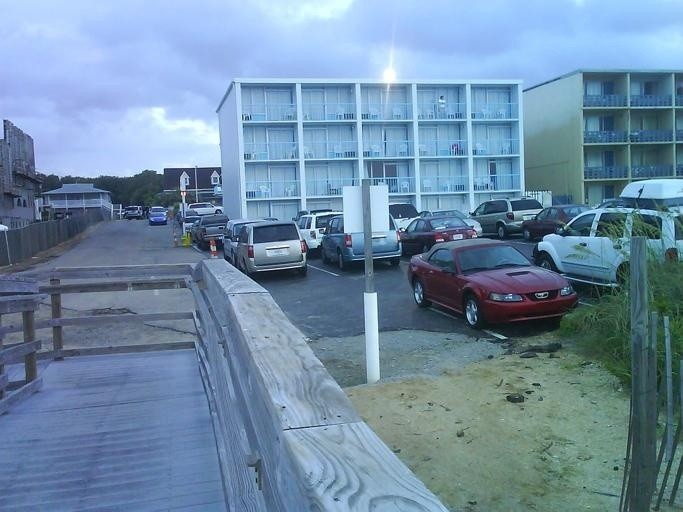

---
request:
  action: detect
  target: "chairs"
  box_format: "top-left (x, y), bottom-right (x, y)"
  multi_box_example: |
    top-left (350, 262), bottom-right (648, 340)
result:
top-left (244, 177), bottom-right (496, 200)
top-left (242, 141), bottom-right (514, 162)
top-left (238, 105), bottom-right (509, 121)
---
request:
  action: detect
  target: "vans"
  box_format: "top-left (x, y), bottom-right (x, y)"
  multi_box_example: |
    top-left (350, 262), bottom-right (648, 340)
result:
top-left (319, 212), bottom-right (402, 271)
top-left (124, 205), bottom-right (143, 220)
top-left (230, 221), bottom-right (306, 280)
top-left (222, 217), bottom-right (270, 268)
top-left (186, 202), bottom-right (224, 216)
top-left (192, 215), bottom-right (229, 250)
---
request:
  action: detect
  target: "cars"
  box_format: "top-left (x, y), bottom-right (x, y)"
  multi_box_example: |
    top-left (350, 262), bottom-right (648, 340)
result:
top-left (185, 216), bottom-right (199, 234)
top-left (408, 238), bottom-right (576, 331)
top-left (388, 203), bottom-right (421, 226)
top-left (520, 205), bottom-right (595, 241)
top-left (595, 200), bottom-right (623, 209)
top-left (397, 209), bottom-right (482, 237)
top-left (146, 206), bottom-right (168, 219)
top-left (148, 212), bottom-right (166, 225)
top-left (467, 196), bottom-right (543, 240)
top-left (395, 215), bottom-right (477, 257)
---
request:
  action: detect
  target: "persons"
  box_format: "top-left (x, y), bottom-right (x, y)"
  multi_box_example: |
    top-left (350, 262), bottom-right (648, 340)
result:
top-left (0, 218), bottom-right (8, 232)
top-left (438, 94), bottom-right (446, 119)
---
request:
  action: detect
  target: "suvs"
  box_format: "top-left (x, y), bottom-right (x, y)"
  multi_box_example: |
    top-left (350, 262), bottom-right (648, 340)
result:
top-left (533, 179), bottom-right (682, 299)
top-left (294, 211), bottom-right (343, 258)
